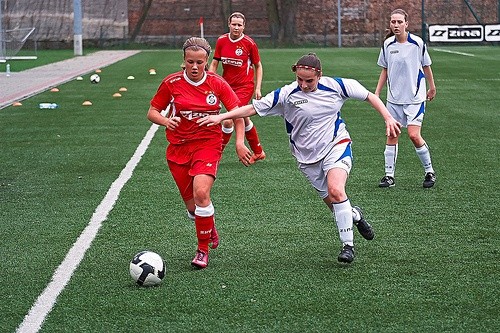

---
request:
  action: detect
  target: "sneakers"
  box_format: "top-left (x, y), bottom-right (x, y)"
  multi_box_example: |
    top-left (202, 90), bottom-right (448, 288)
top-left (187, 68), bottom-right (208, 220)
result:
top-left (209, 215), bottom-right (219, 249)
top-left (378, 176), bottom-right (395, 188)
top-left (250, 150), bottom-right (266, 162)
top-left (423, 172), bottom-right (436, 188)
top-left (352, 206), bottom-right (374, 240)
top-left (191, 249), bottom-right (208, 269)
top-left (338, 244), bottom-right (355, 263)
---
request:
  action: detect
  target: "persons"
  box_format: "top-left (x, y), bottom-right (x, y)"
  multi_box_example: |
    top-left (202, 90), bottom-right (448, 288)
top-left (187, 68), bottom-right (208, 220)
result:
top-left (147, 37), bottom-right (252, 266)
top-left (208, 12), bottom-right (266, 163)
top-left (375, 9), bottom-right (437, 187)
top-left (197, 53), bottom-right (402, 264)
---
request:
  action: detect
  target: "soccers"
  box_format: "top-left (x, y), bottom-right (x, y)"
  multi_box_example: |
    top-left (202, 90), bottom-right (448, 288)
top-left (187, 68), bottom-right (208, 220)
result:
top-left (129, 251), bottom-right (167, 287)
top-left (90, 73), bottom-right (101, 84)
